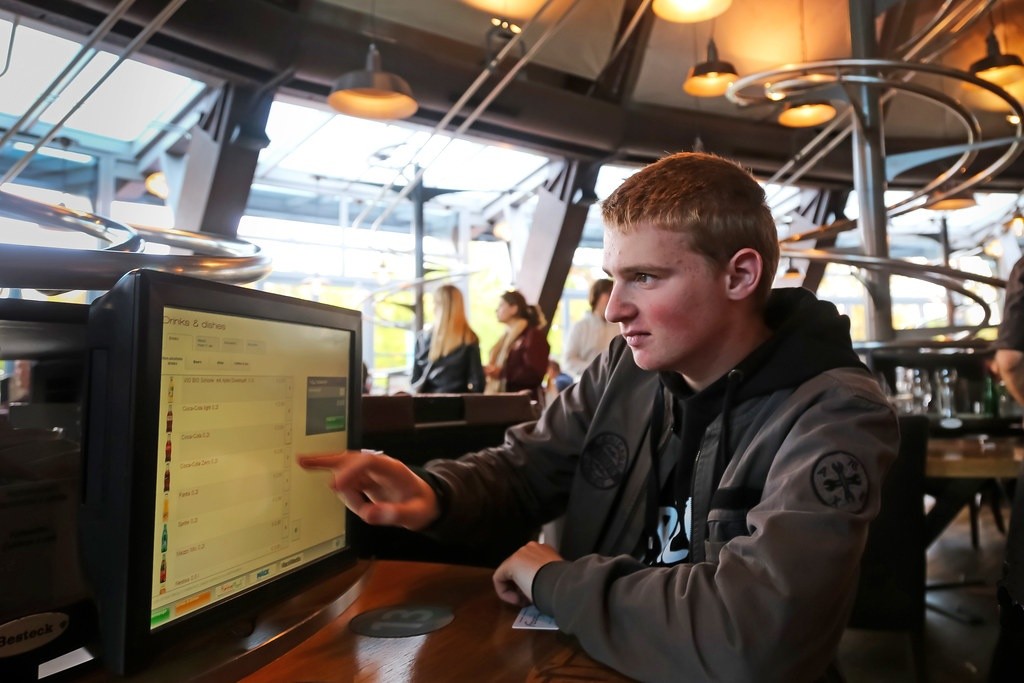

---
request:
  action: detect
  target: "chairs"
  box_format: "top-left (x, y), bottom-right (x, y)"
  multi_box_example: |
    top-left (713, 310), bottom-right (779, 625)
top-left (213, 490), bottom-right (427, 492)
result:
top-left (845, 417), bottom-right (930, 683)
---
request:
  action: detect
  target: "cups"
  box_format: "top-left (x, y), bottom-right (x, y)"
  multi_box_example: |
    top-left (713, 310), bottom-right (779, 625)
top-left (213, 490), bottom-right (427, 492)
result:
top-left (912, 370), bottom-right (932, 415)
top-left (937, 370), bottom-right (957, 416)
top-left (894, 367), bottom-right (913, 395)
top-left (894, 393), bottom-right (914, 412)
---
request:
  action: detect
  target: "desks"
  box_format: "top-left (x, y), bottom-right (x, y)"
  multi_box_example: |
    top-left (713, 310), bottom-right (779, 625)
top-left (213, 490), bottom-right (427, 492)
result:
top-left (180, 558), bottom-right (634, 683)
top-left (854, 323), bottom-right (1002, 352)
top-left (924, 460), bottom-right (1022, 549)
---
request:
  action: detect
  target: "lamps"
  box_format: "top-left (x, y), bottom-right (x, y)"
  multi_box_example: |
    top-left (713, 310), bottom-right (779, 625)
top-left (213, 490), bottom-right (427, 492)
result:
top-left (777, 1), bottom-right (837, 128)
top-left (325, 1), bottom-right (419, 121)
top-left (925, 181), bottom-right (977, 210)
top-left (782, 257), bottom-right (803, 278)
top-left (969, 11), bottom-right (1024, 87)
top-left (681, 18), bottom-right (742, 99)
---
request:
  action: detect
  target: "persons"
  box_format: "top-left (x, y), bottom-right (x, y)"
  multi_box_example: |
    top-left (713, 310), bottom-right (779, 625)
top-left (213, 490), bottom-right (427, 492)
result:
top-left (488, 290), bottom-right (552, 402)
top-left (290, 152), bottom-right (904, 683)
top-left (411, 284), bottom-right (486, 395)
top-left (544, 356), bottom-right (574, 405)
top-left (566, 278), bottom-right (624, 380)
top-left (984, 254), bottom-right (1024, 683)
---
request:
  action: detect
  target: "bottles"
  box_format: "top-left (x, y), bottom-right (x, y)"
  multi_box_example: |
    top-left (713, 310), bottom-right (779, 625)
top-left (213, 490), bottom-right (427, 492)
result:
top-left (999, 381), bottom-right (1012, 420)
top-left (983, 375), bottom-right (999, 420)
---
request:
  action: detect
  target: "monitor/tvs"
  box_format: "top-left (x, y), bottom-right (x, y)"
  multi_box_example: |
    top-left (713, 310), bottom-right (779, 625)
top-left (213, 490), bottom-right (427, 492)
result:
top-left (76, 270), bottom-right (363, 683)
